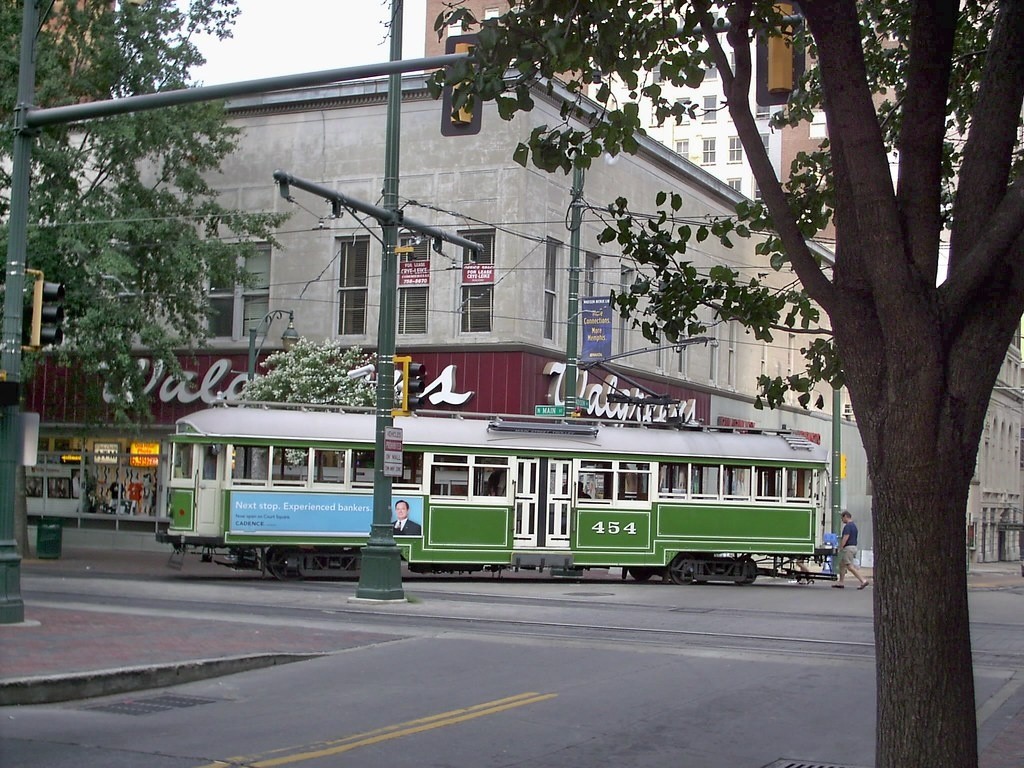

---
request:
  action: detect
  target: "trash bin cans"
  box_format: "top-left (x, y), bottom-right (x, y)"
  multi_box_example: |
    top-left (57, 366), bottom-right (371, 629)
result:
top-left (36, 518), bottom-right (64, 559)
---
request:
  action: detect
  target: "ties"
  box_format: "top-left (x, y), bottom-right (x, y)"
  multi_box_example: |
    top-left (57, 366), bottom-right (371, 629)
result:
top-left (397, 522), bottom-right (401, 531)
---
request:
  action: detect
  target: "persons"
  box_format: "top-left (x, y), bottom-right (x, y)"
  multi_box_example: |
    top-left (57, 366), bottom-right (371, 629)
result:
top-left (391, 500), bottom-right (421, 535)
top-left (486, 460), bottom-right (507, 496)
top-left (578, 481), bottom-right (591, 499)
top-left (203, 445), bottom-right (217, 479)
top-left (831, 511), bottom-right (869, 590)
top-left (795, 559), bottom-right (814, 585)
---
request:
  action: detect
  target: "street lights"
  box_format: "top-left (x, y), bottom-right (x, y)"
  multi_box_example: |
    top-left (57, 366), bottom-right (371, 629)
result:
top-left (247, 308), bottom-right (301, 383)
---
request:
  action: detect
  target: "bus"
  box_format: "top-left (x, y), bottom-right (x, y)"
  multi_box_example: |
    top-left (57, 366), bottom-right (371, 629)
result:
top-left (154, 397), bottom-right (840, 583)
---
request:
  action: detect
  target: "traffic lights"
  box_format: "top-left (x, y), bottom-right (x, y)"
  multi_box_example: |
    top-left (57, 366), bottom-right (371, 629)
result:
top-left (401, 356), bottom-right (427, 414)
top-left (31, 277), bottom-right (65, 347)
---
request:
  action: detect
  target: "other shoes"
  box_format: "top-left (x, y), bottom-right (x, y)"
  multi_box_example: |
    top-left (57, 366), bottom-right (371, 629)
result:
top-left (857, 582), bottom-right (868, 589)
top-left (799, 581), bottom-right (806, 584)
top-left (807, 579), bottom-right (812, 584)
top-left (832, 584), bottom-right (844, 588)
